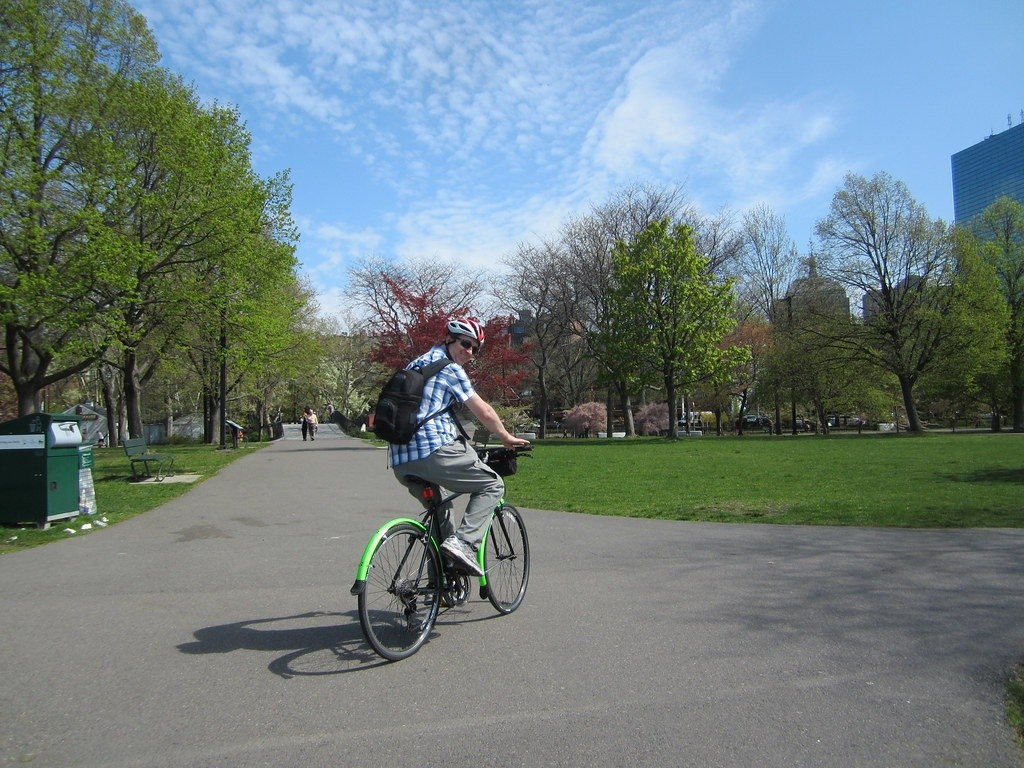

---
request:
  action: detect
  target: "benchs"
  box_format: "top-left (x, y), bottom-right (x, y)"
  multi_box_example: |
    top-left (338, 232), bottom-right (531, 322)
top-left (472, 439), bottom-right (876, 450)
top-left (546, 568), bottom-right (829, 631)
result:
top-left (123, 438), bottom-right (175, 481)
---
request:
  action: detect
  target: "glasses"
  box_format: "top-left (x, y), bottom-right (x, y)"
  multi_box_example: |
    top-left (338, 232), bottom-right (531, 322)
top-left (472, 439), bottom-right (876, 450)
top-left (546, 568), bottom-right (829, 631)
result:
top-left (449, 334), bottom-right (480, 354)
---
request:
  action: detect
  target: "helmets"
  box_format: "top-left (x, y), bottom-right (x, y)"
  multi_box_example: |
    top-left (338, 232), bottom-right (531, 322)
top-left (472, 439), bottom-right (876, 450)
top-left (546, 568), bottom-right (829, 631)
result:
top-left (445, 319), bottom-right (485, 349)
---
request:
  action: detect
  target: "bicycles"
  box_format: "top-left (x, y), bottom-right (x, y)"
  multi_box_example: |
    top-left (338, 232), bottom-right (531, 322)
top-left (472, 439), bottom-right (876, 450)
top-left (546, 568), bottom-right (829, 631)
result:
top-left (350, 428), bottom-right (535, 662)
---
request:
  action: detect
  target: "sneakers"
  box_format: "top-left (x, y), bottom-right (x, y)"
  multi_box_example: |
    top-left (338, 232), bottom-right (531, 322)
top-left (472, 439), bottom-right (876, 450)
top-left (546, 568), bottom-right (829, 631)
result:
top-left (424, 584), bottom-right (467, 606)
top-left (440, 535), bottom-right (485, 577)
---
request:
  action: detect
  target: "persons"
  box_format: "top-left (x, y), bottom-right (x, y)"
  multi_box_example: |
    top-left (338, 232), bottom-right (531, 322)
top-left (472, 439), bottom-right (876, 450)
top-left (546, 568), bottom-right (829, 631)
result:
top-left (96, 431), bottom-right (106, 448)
top-left (386, 317), bottom-right (530, 605)
top-left (300, 409), bottom-right (317, 441)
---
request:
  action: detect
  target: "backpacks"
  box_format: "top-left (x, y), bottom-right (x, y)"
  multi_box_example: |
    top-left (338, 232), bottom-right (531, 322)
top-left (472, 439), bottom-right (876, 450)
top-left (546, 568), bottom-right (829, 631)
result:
top-left (372, 359), bottom-right (459, 444)
